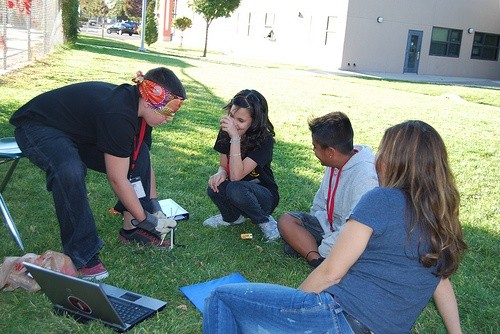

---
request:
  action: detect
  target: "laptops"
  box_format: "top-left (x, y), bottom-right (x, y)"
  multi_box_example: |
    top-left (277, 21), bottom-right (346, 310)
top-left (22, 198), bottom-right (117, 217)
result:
top-left (22, 261), bottom-right (167, 332)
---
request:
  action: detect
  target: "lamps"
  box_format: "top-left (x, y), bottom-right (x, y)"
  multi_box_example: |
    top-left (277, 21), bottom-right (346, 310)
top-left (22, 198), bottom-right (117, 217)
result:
top-left (468, 28), bottom-right (473, 34)
top-left (377, 16), bottom-right (383, 24)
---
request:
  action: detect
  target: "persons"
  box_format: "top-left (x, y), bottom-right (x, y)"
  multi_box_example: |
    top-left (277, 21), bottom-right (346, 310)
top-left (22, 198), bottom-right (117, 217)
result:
top-left (203, 119), bottom-right (463, 334)
top-left (10, 68), bottom-right (188, 280)
top-left (277, 112), bottom-right (380, 267)
top-left (203, 89), bottom-right (281, 240)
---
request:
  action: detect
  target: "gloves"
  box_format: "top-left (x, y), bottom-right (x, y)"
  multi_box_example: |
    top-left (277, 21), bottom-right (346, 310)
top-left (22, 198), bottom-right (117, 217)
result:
top-left (150, 198), bottom-right (167, 220)
top-left (131, 210), bottom-right (177, 240)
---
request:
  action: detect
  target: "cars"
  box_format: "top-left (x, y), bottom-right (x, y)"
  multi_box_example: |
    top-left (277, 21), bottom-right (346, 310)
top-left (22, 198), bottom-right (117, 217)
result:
top-left (106, 21), bottom-right (141, 36)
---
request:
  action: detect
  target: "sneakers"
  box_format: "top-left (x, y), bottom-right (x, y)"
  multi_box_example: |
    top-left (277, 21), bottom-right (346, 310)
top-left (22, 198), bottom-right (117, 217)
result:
top-left (119, 228), bottom-right (171, 248)
top-left (258, 214), bottom-right (280, 240)
top-left (77, 256), bottom-right (109, 280)
top-left (203, 213), bottom-right (244, 228)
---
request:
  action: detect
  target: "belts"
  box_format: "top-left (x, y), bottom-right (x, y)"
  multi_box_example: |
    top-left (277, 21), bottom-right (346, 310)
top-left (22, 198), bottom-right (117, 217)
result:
top-left (341, 310), bottom-right (371, 334)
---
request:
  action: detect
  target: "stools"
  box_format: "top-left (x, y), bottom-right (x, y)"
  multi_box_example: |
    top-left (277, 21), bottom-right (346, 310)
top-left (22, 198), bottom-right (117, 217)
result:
top-left (0, 136), bottom-right (26, 193)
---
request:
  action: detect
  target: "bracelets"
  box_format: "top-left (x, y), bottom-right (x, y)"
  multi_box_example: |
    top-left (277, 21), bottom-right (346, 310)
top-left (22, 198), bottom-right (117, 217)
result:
top-left (229, 136), bottom-right (241, 144)
top-left (229, 153), bottom-right (242, 157)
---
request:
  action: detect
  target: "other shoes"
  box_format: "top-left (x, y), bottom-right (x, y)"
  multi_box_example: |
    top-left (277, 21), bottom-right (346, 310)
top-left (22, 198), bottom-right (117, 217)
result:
top-left (311, 257), bottom-right (325, 268)
top-left (284, 245), bottom-right (304, 259)
